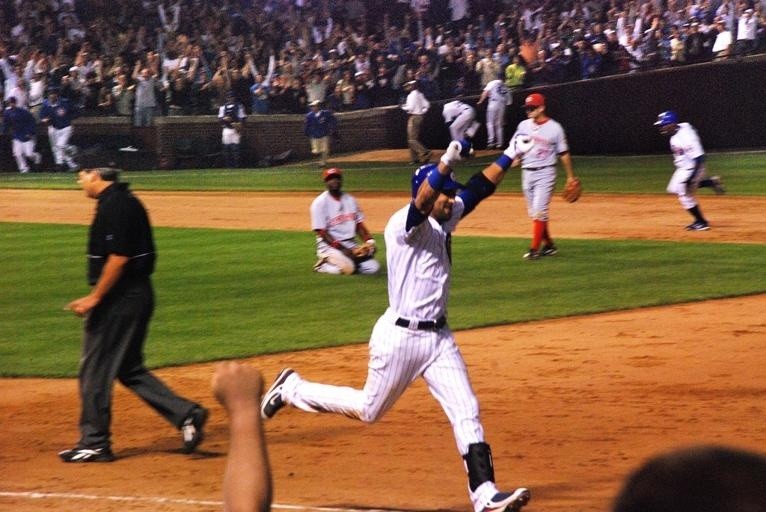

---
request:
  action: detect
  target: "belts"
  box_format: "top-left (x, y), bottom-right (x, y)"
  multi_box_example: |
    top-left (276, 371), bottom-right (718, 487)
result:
top-left (522, 165), bottom-right (556, 171)
top-left (396, 316), bottom-right (445, 331)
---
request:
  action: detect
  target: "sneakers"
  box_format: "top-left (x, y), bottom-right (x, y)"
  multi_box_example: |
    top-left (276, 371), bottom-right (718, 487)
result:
top-left (178, 408), bottom-right (208, 454)
top-left (710, 175), bottom-right (726, 195)
top-left (684, 221), bottom-right (711, 230)
top-left (57, 447), bottom-right (115, 462)
top-left (260, 367), bottom-right (295, 420)
top-left (312, 258), bottom-right (327, 271)
top-left (481, 488), bottom-right (530, 512)
top-left (522, 243), bottom-right (558, 260)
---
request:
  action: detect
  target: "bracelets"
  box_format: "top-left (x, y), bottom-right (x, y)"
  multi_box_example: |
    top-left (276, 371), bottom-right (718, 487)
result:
top-left (330, 240), bottom-right (341, 250)
top-left (366, 238), bottom-right (376, 244)
top-left (423, 167), bottom-right (447, 194)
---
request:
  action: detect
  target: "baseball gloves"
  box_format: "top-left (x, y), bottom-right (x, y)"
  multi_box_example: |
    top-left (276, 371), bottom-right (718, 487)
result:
top-left (352, 239), bottom-right (377, 258)
top-left (561, 177), bottom-right (581, 203)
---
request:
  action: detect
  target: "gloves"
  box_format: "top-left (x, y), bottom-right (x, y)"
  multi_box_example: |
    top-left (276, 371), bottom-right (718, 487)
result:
top-left (440, 139), bottom-right (473, 171)
top-left (497, 133), bottom-right (536, 172)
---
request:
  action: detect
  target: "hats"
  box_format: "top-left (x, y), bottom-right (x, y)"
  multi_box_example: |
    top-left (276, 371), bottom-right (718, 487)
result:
top-left (309, 100), bottom-right (320, 107)
top-left (521, 93), bottom-right (544, 108)
top-left (402, 80), bottom-right (416, 87)
top-left (69, 142), bottom-right (123, 173)
top-left (322, 168), bottom-right (342, 179)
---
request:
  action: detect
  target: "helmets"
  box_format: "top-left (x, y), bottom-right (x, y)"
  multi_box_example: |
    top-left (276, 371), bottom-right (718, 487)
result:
top-left (653, 111), bottom-right (676, 126)
top-left (411, 163), bottom-right (465, 198)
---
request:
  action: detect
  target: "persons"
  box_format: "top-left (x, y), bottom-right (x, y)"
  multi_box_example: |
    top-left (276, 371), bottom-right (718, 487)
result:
top-left (652, 110), bottom-right (726, 232)
top-left (610, 442), bottom-right (766, 512)
top-left (257, 134), bottom-right (532, 512)
top-left (56, 140), bottom-right (209, 462)
top-left (212, 358), bottom-right (273, 512)
top-left (310, 167), bottom-right (382, 276)
top-left (1, 1), bottom-right (766, 172)
top-left (509, 94), bottom-right (574, 260)
